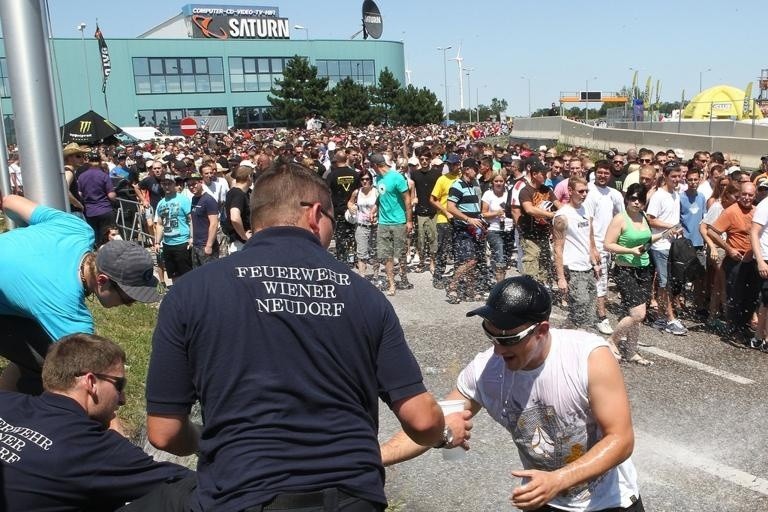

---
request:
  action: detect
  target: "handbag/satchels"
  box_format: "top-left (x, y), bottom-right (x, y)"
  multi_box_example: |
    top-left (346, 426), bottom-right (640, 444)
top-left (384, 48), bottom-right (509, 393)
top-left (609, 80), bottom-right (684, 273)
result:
top-left (466, 217), bottom-right (489, 242)
top-left (343, 206), bottom-right (360, 225)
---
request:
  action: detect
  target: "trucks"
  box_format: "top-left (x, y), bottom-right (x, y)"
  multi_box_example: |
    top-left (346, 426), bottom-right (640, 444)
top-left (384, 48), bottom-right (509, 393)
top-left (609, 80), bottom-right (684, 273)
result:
top-left (120, 126), bottom-right (164, 140)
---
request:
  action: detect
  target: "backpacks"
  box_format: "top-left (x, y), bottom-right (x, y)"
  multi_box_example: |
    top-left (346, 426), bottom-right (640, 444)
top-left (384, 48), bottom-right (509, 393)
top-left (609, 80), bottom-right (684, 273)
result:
top-left (667, 228), bottom-right (706, 294)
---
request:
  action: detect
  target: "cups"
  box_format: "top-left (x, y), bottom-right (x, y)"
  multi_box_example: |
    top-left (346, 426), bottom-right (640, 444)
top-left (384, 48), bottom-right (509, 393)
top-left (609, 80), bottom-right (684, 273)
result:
top-left (436, 399), bottom-right (469, 463)
top-left (113, 235), bottom-right (123, 240)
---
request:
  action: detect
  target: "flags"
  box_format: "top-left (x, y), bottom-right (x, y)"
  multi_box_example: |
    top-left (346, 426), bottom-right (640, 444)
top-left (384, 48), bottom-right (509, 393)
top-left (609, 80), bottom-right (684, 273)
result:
top-left (95, 27), bottom-right (111, 94)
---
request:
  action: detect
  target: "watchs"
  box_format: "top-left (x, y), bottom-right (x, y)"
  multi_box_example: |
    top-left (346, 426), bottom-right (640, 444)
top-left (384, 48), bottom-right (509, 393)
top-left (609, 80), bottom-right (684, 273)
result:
top-left (434, 422), bottom-right (454, 451)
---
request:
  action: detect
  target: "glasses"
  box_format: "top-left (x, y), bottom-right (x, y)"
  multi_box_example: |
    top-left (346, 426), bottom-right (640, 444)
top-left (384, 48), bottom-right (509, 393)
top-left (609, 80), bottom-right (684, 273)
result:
top-left (94, 374), bottom-right (128, 393)
top-left (698, 159), bottom-right (708, 163)
top-left (481, 320), bottom-right (544, 346)
top-left (613, 160), bottom-right (624, 165)
top-left (639, 158), bottom-right (651, 163)
top-left (360, 177), bottom-right (370, 182)
top-left (630, 196), bottom-right (645, 204)
top-left (574, 189), bottom-right (590, 194)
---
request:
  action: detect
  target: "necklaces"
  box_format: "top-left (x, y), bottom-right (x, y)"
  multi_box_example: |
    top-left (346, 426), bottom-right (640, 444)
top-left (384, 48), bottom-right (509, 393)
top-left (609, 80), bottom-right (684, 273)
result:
top-left (79, 248), bottom-right (100, 304)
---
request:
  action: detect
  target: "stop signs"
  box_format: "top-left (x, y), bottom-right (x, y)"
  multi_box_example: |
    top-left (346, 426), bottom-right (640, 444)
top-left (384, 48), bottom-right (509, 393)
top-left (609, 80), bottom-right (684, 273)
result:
top-left (180, 117), bottom-right (198, 137)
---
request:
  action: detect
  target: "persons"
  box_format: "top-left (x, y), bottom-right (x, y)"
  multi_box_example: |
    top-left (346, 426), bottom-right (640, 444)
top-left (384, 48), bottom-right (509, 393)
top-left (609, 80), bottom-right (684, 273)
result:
top-left (0, 192), bottom-right (163, 394)
top-left (0, 332), bottom-right (201, 511)
top-left (145, 162), bottom-right (471, 510)
top-left (378, 275), bottom-right (648, 511)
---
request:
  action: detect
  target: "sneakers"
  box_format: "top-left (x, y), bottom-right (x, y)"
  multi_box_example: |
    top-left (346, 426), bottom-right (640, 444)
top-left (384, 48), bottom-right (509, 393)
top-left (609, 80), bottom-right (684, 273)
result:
top-left (662, 319), bottom-right (690, 336)
top-left (595, 318), bottom-right (615, 336)
top-left (335, 249), bottom-right (481, 305)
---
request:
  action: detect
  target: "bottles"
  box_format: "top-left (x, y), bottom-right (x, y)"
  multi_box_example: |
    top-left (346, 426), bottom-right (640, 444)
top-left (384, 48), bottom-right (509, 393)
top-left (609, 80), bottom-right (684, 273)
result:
top-left (475, 216), bottom-right (482, 236)
top-left (351, 203), bottom-right (358, 222)
top-left (347, 246), bottom-right (354, 264)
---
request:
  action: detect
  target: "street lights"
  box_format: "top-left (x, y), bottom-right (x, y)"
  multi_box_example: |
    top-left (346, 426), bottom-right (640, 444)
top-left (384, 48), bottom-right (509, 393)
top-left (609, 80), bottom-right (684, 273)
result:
top-left (437, 46), bottom-right (482, 128)
top-left (520, 76), bottom-right (531, 118)
top-left (629, 67), bottom-right (639, 100)
top-left (699, 69), bottom-right (712, 92)
top-left (399, 31), bottom-right (411, 88)
top-left (77, 22), bottom-right (93, 111)
top-left (585, 76), bottom-right (599, 122)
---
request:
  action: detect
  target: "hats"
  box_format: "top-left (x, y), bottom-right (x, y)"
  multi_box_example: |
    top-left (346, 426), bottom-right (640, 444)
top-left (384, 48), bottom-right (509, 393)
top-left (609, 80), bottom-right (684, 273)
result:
top-left (94, 238), bottom-right (164, 304)
top-left (63, 142), bottom-right (92, 158)
top-left (160, 173), bottom-right (176, 184)
top-left (466, 275), bottom-right (552, 331)
top-left (442, 155), bottom-right (462, 164)
top-left (112, 145), bottom-right (257, 172)
top-left (181, 172), bottom-right (203, 181)
top-left (756, 155), bottom-right (768, 190)
top-left (530, 162), bottom-right (554, 173)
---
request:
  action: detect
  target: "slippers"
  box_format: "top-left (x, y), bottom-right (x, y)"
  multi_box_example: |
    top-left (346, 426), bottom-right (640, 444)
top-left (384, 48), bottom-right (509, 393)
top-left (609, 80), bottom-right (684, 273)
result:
top-left (605, 339), bottom-right (624, 364)
top-left (624, 355), bottom-right (652, 366)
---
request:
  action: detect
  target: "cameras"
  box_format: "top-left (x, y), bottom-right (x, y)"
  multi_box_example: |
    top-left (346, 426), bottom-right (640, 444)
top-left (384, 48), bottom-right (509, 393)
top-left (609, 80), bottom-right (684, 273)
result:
top-left (479, 213), bottom-right (492, 227)
